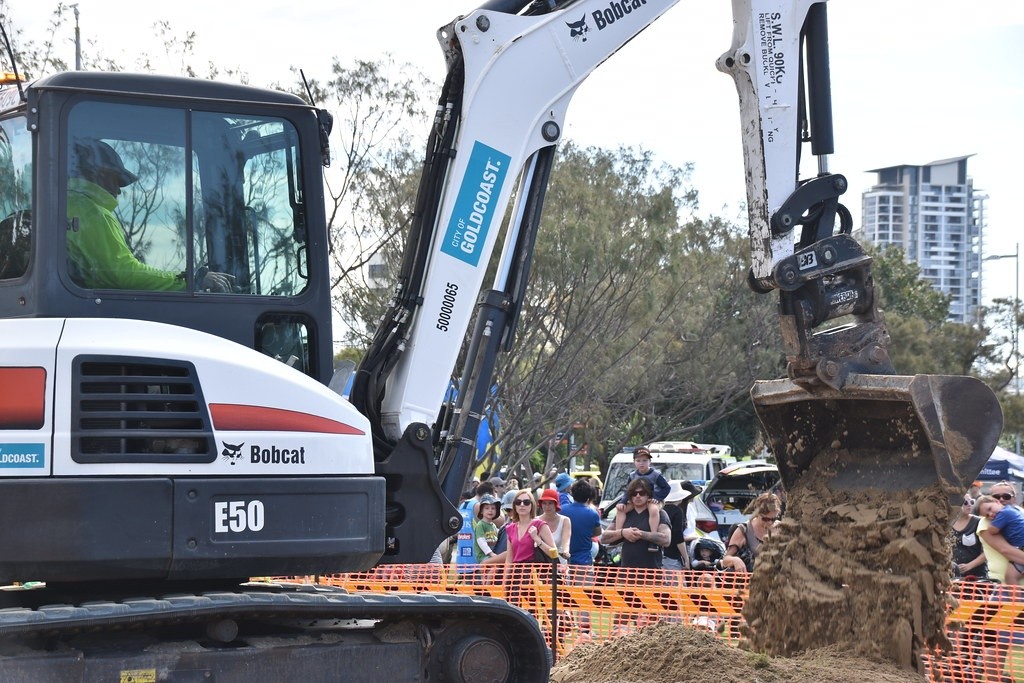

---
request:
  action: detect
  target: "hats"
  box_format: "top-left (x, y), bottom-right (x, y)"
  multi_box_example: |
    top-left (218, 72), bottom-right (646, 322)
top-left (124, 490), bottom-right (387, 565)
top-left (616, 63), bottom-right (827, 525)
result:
top-left (477, 494), bottom-right (500, 520)
top-left (681, 481), bottom-right (702, 502)
top-left (533, 472), bottom-right (542, 481)
top-left (972, 480), bottom-right (982, 487)
top-left (489, 477), bottom-right (507, 488)
top-left (664, 480), bottom-right (692, 502)
top-left (555, 473), bottom-right (576, 491)
top-left (473, 477), bottom-right (479, 483)
top-left (633, 446), bottom-right (653, 458)
top-left (538, 489), bottom-right (562, 511)
top-left (501, 490), bottom-right (518, 509)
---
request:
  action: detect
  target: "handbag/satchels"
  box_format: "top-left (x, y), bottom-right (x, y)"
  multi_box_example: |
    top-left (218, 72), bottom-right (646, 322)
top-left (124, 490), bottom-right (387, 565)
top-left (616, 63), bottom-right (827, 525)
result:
top-left (724, 524), bottom-right (754, 588)
top-left (533, 541), bottom-right (560, 578)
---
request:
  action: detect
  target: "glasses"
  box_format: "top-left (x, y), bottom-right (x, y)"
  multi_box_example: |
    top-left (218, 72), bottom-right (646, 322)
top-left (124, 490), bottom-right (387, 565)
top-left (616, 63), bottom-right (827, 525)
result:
top-left (515, 499), bottom-right (530, 505)
top-left (500, 485), bottom-right (503, 487)
top-left (505, 508), bottom-right (511, 512)
top-left (760, 515), bottom-right (779, 522)
top-left (992, 493), bottom-right (1014, 500)
top-left (964, 499), bottom-right (975, 505)
top-left (631, 490), bottom-right (646, 496)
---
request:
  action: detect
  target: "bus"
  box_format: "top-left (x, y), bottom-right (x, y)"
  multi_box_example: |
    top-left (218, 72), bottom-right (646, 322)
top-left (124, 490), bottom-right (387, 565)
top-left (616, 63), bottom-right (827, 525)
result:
top-left (977, 443), bottom-right (1024, 513)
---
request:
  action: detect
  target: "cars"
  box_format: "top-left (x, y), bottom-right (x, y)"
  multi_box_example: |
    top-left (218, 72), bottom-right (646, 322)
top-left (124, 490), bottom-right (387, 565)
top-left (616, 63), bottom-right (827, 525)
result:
top-left (568, 440), bottom-right (783, 542)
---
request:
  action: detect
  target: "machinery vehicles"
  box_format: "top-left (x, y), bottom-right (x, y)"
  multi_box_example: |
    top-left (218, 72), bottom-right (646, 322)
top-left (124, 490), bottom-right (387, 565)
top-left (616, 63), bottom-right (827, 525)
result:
top-left (0, 0), bottom-right (1007, 683)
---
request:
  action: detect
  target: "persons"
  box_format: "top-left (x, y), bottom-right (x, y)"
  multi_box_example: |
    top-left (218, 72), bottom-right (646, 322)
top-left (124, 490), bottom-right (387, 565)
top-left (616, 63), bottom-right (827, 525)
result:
top-left (431, 472), bottom-right (1024, 683)
top-left (606, 446), bottom-right (671, 551)
top-left (66, 138), bottom-right (235, 294)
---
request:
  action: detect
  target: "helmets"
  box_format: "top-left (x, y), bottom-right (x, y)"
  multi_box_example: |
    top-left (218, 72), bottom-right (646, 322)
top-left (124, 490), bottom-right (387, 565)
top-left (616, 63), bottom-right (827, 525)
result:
top-left (71, 137), bottom-right (138, 187)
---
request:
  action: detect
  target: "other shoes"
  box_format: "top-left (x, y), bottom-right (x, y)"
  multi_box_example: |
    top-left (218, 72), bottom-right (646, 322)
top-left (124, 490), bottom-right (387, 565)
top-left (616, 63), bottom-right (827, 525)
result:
top-left (647, 543), bottom-right (659, 552)
top-left (607, 539), bottom-right (624, 547)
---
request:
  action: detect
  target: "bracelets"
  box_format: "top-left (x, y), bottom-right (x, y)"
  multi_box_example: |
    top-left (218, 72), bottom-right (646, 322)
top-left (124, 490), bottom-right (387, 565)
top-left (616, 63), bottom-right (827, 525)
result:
top-left (537, 541), bottom-right (543, 547)
top-left (620, 528), bottom-right (624, 538)
